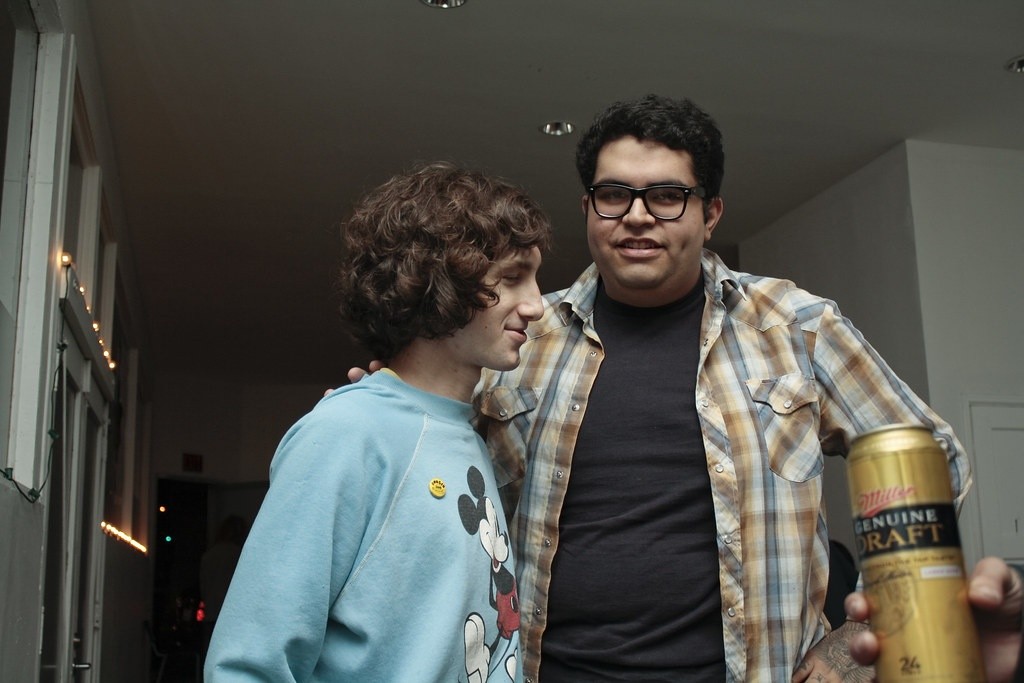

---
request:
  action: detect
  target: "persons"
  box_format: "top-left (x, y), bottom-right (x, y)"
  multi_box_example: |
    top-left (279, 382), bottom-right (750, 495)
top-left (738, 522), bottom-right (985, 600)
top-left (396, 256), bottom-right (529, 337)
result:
top-left (204, 160), bottom-right (545, 683)
top-left (199, 514), bottom-right (245, 680)
top-left (324, 96), bottom-right (971, 683)
top-left (844, 557), bottom-right (1024, 683)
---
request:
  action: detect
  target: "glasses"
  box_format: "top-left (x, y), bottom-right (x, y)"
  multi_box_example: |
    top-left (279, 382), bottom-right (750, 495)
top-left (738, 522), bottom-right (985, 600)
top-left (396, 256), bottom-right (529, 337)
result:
top-left (586, 183), bottom-right (706, 221)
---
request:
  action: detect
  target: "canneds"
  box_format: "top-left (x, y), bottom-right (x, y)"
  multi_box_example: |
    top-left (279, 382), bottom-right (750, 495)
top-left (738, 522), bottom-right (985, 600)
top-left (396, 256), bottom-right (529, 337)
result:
top-left (845, 423), bottom-right (987, 683)
